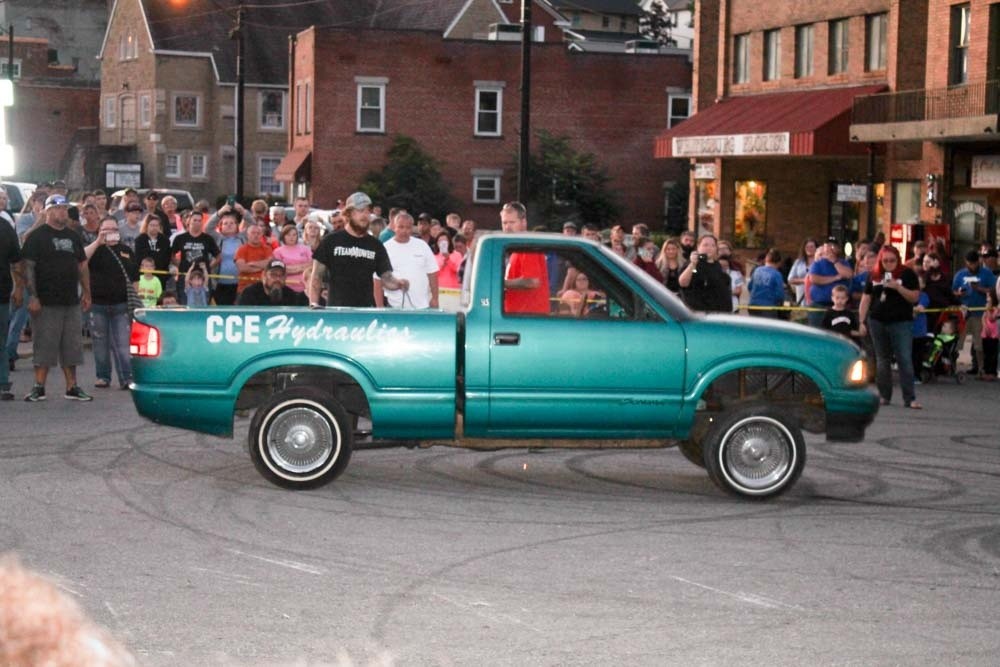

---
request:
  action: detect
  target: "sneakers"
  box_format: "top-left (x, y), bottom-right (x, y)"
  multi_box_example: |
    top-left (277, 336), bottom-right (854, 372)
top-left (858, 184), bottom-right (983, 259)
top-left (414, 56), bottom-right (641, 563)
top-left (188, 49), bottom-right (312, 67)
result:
top-left (23, 382), bottom-right (48, 400)
top-left (63, 384), bottom-right (93, 402)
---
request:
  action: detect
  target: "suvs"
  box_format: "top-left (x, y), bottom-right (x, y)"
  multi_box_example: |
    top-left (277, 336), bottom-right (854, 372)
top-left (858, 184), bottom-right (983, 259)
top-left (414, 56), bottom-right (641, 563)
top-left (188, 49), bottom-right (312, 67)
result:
top-left (0, 180), bottom-right (37, 225)
top-left (109, 188), bottom-right (195, 217)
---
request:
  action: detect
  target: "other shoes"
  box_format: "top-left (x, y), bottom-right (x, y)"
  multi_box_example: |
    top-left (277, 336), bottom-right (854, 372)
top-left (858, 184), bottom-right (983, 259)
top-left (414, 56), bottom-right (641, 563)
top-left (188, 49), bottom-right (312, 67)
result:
top-left (878, 396), bottom-right (890, 406)
top-left (0, 392), bottom-right (15, 404)
top-left (976, 375), bottom-right (997, 383)
top-left (94, 376), bottom-right (111, 387)
top-left (904, 401), bottom-right (923, 409)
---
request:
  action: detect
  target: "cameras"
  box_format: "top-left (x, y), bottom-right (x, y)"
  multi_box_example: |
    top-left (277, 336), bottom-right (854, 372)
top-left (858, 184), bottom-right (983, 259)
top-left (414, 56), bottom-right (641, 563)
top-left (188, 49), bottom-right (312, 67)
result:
top-left (698, 253), bottom-right (707, 262)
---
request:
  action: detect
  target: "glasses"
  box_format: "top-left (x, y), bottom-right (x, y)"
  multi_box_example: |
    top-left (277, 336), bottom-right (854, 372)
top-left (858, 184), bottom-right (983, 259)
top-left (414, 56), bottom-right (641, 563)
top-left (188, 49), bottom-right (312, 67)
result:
top-left (881, 259), bottom-right (898, 264)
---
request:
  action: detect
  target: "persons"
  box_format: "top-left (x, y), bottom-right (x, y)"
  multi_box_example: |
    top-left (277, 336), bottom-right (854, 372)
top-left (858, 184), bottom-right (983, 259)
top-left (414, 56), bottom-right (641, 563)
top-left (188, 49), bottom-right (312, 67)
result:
top-left (0, 180), bottom-right (182, 401)
top-left (905, 242), bottom-right (1000, 384)
top-left (859, 246), bottom-right (923, 410)
top-left (748, 236), bottom-right (897, 371)
top-left (373, 215), bottom-right (439, 308)
top-left (169, 196), bottom-right (348, 306)
top-left (535, 221), bottom-right (746, 318)
top-left (309, 193), bottom-right (409, 307)
top-left (368, 207), bottom-right (475, 290)
top-left (21, 194), bottom-right (94, 402)
top-left (80, 216), bottom-right (139, 388)
top-left (501, 204), bottom-right (549, 314)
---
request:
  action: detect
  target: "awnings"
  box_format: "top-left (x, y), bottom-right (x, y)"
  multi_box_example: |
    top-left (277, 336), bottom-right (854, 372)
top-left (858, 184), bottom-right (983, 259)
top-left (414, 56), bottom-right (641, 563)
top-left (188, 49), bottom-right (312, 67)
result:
top-left (274, 152), bottom-right (311, 183)
top-left (656, 84), bottom-right (886, 156)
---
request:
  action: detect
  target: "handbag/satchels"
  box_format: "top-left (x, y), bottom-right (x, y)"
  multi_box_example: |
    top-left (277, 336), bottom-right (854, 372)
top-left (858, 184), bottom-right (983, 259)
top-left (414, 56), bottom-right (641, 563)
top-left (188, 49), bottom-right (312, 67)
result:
top-left (126, 281), bottom-right (145, 325)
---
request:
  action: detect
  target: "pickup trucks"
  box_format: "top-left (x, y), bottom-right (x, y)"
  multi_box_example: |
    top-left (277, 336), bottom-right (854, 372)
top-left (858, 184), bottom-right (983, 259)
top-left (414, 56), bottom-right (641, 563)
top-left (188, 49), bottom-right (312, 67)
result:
top-left (127, 231), bottom-right (882, 505)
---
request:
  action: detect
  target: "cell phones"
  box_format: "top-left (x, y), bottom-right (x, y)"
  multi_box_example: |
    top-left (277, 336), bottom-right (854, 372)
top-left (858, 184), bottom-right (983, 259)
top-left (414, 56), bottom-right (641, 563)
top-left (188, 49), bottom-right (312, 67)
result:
top-left (439, 241), bottom-right (448, 253)
top-left (885, 272), bottom-right (892, 281)
top-left (106, 233), bottom-right (120, 241)
top-left (228, 196), bottom-right (235, 206)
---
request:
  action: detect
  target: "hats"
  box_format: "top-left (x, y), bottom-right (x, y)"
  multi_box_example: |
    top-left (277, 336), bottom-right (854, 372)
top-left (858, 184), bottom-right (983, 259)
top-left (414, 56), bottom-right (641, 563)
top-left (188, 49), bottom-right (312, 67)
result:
top-left (125, 188), bottom-right (137, 195)
top-left (369, 213), bottom-right (384, 223)
top-left (562, 220), bottom-right (577, 229)
top-left (824, 235), bottom-right (840, 245)
top-left (265, 258), bottom-right (286, 272)
top-left (45, 194), bottom-right (71, 211)
top-left (144, 189), bottom-right (158, 198)
top-left (982, 249), bottom-right (998, 259)
top-left (966, 252), bottom-right (980, 263)
top-left (415, 213), bottom-right (433, 223)
top-left (128, 202), bottom-right (144, 212)
top-left (345, 191), bottom-right (373, 209)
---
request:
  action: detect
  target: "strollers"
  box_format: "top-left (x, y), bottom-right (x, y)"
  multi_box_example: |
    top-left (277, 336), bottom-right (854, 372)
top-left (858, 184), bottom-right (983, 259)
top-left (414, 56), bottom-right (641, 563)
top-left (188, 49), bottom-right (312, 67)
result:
top-left (914, 303), bottom-right (973, 386)
